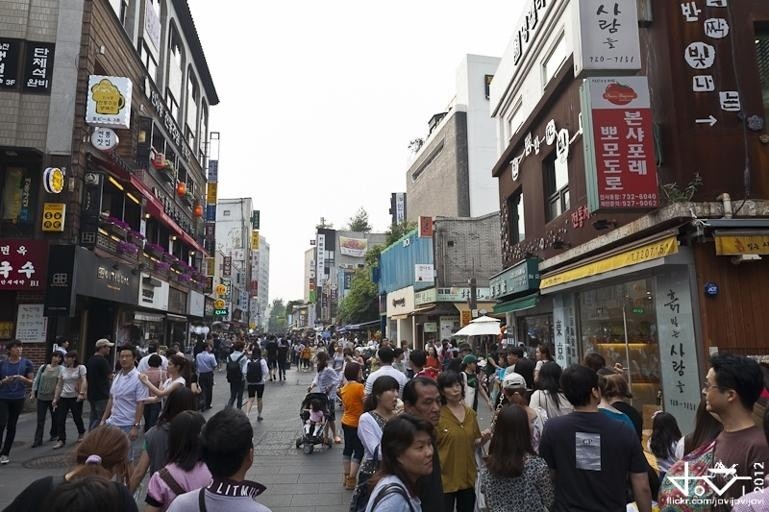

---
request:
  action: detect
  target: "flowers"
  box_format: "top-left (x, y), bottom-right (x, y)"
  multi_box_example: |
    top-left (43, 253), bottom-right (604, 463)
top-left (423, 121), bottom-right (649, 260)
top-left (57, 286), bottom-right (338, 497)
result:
top-left (100, 213), bottom-right (210, 292)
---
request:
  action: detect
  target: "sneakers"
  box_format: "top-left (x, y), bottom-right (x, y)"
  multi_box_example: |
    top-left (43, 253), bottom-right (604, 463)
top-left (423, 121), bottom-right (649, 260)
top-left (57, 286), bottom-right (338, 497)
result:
top-left (0, 454), bottom-right (10, 464)
top-left (334, 436), bottom-right (341, 445)
top-left (52, 440), bottom-right (65, 449)
top-left (75, 434), bottom-right (86, 443)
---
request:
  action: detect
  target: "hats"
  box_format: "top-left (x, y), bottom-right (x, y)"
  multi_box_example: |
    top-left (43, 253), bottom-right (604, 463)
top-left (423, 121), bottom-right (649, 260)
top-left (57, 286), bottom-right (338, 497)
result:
top-left (461, 354), bottom-right (482, 366)
top-left (458, 342), bottom-right (473, 353)
top-left (502, 371), bottom-right (528, 390)
top-left (95, 338), bottom-right (115, 349)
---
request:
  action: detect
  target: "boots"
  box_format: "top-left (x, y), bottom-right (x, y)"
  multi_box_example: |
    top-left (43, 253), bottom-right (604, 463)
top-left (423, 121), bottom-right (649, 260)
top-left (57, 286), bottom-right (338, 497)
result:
top-left (342, 472), bottom-right (359, 491)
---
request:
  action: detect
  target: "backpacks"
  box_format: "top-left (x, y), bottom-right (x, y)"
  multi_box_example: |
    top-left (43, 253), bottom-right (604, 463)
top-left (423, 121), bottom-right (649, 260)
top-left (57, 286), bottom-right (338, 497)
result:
top-left (246, 357), bottom-right (263, 383)
top-left (226, 353), bottom-right (245, 383)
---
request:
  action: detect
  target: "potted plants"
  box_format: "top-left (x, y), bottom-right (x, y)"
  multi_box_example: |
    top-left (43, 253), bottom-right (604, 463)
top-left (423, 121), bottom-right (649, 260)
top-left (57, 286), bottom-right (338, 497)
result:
top-left (181, 189), bottom-right (196, 208)
top-left (158, 158), bottom-right (178, 183)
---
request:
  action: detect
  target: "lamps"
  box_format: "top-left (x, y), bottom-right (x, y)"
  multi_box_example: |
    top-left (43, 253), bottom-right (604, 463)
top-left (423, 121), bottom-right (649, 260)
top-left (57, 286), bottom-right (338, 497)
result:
top-left (550, 240), bottom-right (572, 250)
top-left (591, 218), bottom-right (617, 230)
top-left (737, 253), bottom-right (763, 265)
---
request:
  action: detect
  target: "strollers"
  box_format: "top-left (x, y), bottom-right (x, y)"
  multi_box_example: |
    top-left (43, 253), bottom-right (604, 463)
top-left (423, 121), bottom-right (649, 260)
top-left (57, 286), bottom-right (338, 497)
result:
top-left (294, 386), bottom-right (335, 453)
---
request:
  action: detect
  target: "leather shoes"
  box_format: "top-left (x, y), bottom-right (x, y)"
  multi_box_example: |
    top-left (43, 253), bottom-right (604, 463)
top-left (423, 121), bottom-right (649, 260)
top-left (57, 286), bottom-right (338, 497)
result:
top-left (49, 434), bottom-right (60, 442)
top-left (31, 440), bottom-right (43, 449)
top-left (257, 415), bottom-right (264, 422)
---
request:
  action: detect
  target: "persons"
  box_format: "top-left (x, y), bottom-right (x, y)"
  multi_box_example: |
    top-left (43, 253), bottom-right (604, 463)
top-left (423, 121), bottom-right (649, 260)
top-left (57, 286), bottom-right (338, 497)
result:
top-left (0, 327), bottom-right (769, 511)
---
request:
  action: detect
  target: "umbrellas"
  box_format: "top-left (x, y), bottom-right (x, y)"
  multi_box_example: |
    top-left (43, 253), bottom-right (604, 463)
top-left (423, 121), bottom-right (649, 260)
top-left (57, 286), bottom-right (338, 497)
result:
top-left (452, 316), bottom-right (501, 357)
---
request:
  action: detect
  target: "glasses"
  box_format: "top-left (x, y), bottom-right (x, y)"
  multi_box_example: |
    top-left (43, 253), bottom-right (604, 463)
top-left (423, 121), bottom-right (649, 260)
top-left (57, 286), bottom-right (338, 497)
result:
top-left (703, 380), bottom-right (728, 392)
